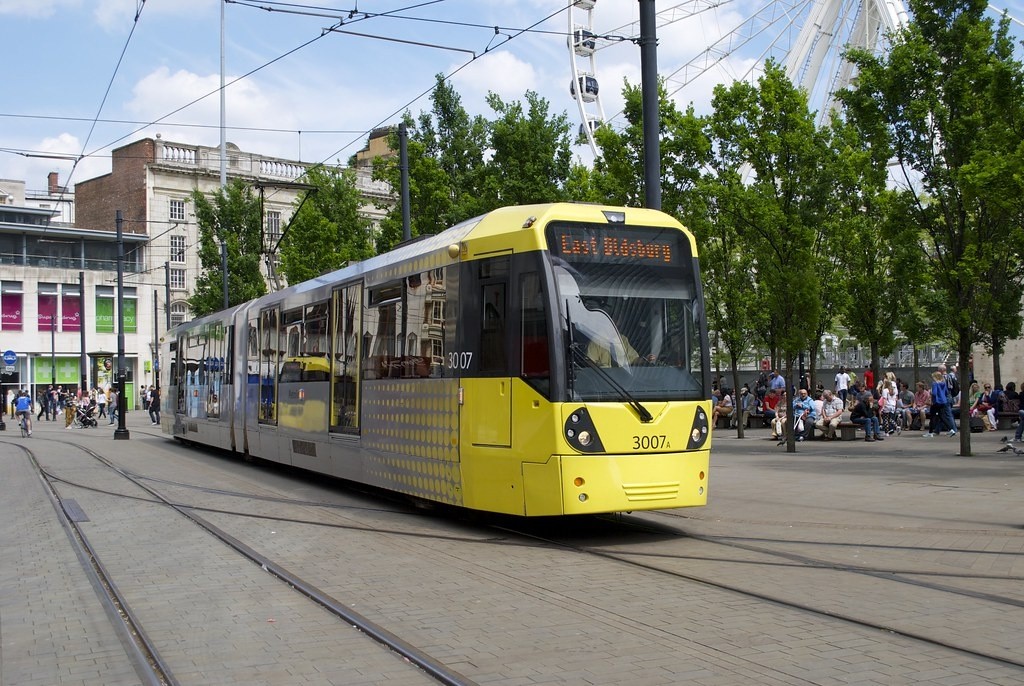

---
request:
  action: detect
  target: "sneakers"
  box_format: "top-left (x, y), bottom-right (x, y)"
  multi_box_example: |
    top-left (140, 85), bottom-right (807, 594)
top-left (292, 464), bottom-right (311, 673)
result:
top-left (923, 433), bottom-right (933, 438)
top-left (946, 429), bottom-right (955, 437)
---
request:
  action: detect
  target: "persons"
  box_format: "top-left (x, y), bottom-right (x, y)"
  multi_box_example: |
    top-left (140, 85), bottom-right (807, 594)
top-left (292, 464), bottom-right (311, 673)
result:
top-left (11, 385), bottom-right (118, 436)
top-left (711, 365), bottom-right (1023, 442)
top-left (149, 385), bottom-right (159, 425)
top-left (139, 385), bottom-right (151, 410)
top-left (586, 308), bottom-right (656, 368)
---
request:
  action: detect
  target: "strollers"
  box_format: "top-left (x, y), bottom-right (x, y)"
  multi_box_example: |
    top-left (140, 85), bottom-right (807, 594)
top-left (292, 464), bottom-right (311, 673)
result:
top-left (73, 405), bottom-right (97, 429)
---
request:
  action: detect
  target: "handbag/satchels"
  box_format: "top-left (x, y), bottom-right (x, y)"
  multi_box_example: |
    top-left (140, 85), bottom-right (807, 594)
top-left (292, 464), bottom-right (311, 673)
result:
top-left (978, 404), bottom-right (992, 411)
top-left (909, 413), bottom-right (921, 430)
top-left (797, 419), bottom-right (804, 432)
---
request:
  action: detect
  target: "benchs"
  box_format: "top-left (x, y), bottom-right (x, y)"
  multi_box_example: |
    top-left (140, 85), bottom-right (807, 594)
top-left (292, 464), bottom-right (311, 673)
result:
top-left (807, 412), bottom-right (873, 441)
top-left (876, 412), bottom-right (1021, 429)
top-left (717, 414), bottom-right (767, 428)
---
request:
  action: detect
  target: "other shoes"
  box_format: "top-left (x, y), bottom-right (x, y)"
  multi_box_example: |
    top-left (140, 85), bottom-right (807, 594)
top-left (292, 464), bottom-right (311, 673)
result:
top-left (873, 435), bottom-right (884, 440)
top-left (152, 423), bottom-right (156, 426)
top-left (743, 426), bottom-right (747, 429)
top-left (1008, 438), bottom-right (1021, 443)
top-left (729, 426), bottom-right (735, 429)
top-left (27, 430), bottom-right (34, 435)
top-left (833, 431), bottom-right (837, 440)
top-left (881, 433), bottom-right (889, 437)
top-left (771, 437), bottom-right (778, 441)
top-left (865, 435), bottom-right (875, 441)
top-left (799, 436), bottom-right (804, 441)
top-left (896, 427), bottom-right (901, 436)
top-left (75, 425), bottom-right (82, 429)
top-left (65, 426), bottom-right (72, 429)
top-left (19, 422), bottom-right (21, 426)
top-left (108, 423), bottom-right (114, 426)
top-left (824, 437), bottom-right (833, 441)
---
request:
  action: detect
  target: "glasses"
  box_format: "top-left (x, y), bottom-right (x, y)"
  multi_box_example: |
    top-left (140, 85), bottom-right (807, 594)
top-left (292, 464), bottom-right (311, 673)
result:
top-left (984, 387), bottom-right (990, 389)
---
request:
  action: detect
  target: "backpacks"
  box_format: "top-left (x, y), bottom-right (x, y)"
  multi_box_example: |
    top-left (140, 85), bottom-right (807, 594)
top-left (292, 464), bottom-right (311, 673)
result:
top-left (948, 374), bottom-right (960, 397)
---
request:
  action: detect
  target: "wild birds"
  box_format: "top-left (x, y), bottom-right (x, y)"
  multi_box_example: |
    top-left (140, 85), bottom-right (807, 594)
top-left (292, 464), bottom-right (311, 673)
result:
top-left (1000, 436), bottom-right (1008, 444)
top-left (997, 443), bottom-right (1024, 456)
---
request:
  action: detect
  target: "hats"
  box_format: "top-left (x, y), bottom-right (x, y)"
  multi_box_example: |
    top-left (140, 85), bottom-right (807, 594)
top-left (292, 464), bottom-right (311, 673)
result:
top-left (740, 388), bottom-right (748, 394)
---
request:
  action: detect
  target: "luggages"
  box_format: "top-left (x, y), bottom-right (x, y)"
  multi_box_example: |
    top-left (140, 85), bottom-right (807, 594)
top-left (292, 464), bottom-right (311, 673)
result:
top-left (969, 411), bottom-right (984, 433)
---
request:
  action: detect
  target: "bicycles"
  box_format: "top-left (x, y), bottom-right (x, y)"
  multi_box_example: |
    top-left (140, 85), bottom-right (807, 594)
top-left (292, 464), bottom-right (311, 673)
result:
top-left (20, 410), bottom-right (34, 438)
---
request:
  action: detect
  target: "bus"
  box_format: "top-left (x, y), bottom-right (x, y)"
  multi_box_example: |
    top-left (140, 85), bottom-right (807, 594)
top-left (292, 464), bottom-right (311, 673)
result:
top-left (157, 184), bottom-right (714, 518)
